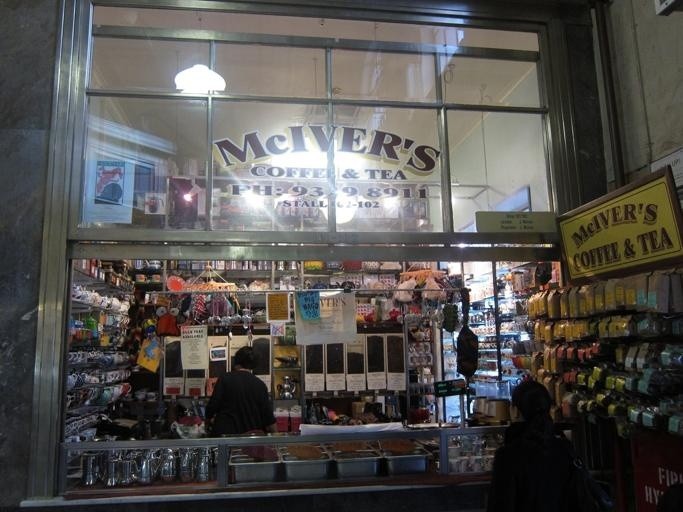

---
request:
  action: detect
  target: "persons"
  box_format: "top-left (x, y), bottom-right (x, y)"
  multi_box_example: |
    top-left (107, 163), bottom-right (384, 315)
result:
top-left (487, 381), bottom-right (577, 512)
top-left (205, 346), bottom-right (277, 437)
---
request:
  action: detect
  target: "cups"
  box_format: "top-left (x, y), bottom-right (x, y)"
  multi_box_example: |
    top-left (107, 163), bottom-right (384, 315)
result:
top-left (68, 351), bottom-right (132, 404)
top-left (448, 440), bottom-right (497, 474)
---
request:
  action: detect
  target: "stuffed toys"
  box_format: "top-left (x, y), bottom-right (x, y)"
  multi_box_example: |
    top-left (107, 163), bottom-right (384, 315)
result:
top-left (156, 306), bottom-right (179, 336)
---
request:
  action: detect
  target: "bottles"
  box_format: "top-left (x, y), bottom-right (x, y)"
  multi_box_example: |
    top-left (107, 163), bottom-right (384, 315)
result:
top-left (73, 316), bottom-right (97, 341)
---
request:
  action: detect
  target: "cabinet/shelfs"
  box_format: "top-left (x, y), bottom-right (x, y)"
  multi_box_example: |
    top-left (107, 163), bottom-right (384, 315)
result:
top-left (64, 177), bottom-right (444, 444)
top-left (446, 262), bottom-right (683, 443)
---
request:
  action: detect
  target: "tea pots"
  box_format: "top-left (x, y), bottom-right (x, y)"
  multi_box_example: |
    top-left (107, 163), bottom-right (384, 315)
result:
top-left (96, 449), bottom-right (214, 485)
top-left (410, 330), bottom-right (428, 342)
